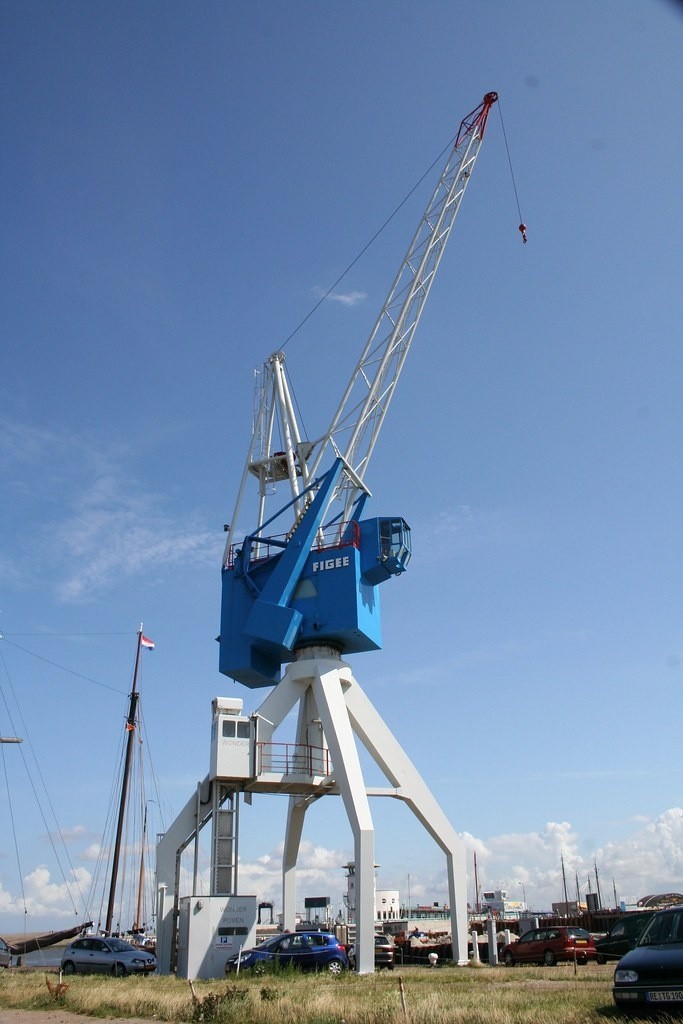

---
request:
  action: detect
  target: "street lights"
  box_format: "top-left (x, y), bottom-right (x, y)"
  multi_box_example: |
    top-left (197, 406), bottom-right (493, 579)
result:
top-left (518, 881), bottom-right (525, 902)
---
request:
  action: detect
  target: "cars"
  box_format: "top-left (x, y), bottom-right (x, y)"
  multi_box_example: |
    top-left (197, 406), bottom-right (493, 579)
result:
top-left (499, 926), bottom-right (597, 965)
top-left (61, 936), bottom-right (157, 976)
top-left (0, 936), bottom-right (12, 968)
top-left (348, 935), bottom-right (394, 969)
top-left (595, 911), bottom-right (658, 964)
top-left (225, 931), bottom-right (349, 974)
top-left (612, 907), bottom-right (683, 1007)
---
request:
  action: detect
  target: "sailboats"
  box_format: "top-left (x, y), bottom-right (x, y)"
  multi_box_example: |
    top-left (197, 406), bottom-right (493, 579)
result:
top-left (0, 737), bottom-right (94, 955)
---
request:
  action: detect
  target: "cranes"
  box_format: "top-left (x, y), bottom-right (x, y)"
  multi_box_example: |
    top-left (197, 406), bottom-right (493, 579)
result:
top-left (155, 87), bottom-right (498, 982)
